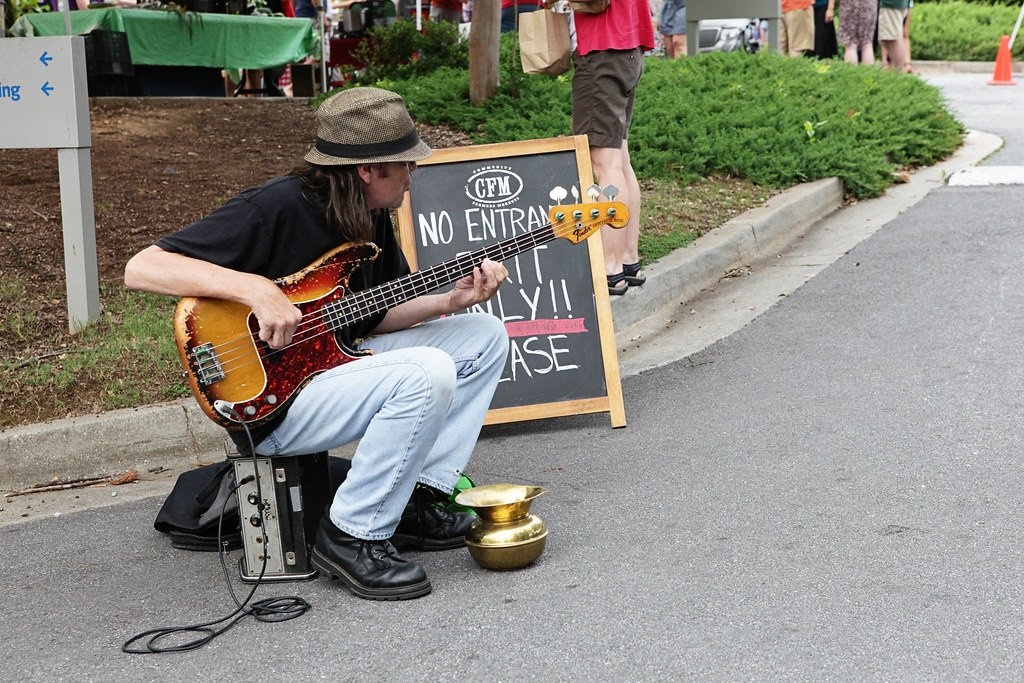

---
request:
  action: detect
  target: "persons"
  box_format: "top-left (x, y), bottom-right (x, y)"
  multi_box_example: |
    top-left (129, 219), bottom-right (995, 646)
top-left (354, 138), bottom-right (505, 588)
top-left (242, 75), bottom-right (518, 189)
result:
top-left (124, 87), bottom-right (510, 601)
top-left (752, 0), bottom-right (913, 72)
top-left (41, 0), bottom-right (549, 98)
top-left (571, 0), bottom-right (655, 296)
top-left (645, 0), bottom-right (688, 59)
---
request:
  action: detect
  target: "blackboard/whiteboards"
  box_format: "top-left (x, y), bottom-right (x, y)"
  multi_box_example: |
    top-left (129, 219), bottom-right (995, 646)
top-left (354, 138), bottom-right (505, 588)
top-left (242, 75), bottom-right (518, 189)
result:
top-left (394, 134), bottom-right (626, 428)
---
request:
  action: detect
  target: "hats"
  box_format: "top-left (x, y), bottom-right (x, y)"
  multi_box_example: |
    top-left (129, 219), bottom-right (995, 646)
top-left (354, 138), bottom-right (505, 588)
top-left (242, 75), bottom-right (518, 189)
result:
top-left (304, 86), bottom-right (432, 166)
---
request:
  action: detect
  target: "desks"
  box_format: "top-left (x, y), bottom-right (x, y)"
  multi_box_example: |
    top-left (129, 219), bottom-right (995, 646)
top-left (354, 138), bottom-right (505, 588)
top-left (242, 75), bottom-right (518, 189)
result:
top-left (10, 9), bottom-right (321, 96)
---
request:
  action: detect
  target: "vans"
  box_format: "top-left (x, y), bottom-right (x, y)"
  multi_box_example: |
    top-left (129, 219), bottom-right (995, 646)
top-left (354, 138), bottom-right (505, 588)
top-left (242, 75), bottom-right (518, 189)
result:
top-left (697, 18), bottom-right (752, 55)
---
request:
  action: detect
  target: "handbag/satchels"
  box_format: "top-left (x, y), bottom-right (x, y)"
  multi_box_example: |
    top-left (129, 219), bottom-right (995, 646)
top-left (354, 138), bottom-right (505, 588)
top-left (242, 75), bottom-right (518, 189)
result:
top-left (153, 456), bottom-right (352, 552)
top-left (518, 0), bottom-right (571, 75)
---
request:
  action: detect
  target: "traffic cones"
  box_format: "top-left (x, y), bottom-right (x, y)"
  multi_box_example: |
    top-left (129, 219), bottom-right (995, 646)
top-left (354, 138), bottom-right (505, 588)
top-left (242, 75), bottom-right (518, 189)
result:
top-left (987, 35), bottom-right (1019, 86)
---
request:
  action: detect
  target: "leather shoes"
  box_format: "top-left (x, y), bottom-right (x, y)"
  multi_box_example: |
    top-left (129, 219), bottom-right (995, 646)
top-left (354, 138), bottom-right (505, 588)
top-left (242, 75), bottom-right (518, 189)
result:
top-left (308, 504), bottom-right (432, 600)
top-left (387, 504), bottom-right (478, 553)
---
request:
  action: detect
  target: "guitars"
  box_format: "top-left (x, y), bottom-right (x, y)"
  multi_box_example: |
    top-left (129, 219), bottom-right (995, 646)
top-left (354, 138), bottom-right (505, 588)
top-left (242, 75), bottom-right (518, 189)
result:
top-left (174, 183), bottom-right (631, 432)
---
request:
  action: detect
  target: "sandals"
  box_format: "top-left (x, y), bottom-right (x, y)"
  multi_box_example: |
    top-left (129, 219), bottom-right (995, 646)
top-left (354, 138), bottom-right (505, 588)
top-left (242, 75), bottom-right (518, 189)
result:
top-left (606, 268), bottom-right (629, 295)
top-left (622, 259), bottom-right (646, 286)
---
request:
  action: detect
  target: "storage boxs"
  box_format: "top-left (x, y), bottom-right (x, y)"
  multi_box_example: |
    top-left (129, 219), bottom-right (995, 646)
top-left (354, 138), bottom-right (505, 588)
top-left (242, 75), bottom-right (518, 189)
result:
top-left (78, 29), bottom-right (134, 96)
